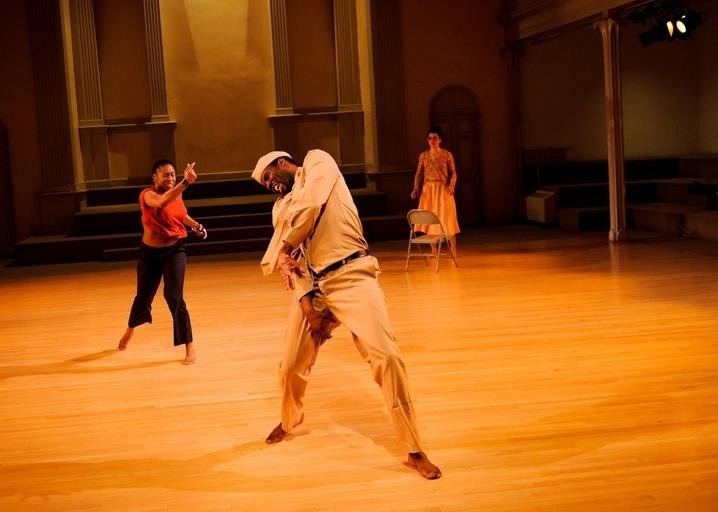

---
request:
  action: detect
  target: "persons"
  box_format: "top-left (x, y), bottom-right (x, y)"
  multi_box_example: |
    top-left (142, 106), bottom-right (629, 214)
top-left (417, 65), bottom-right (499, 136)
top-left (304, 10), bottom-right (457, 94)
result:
top-left (251, 148), bottom-right (443, 481)
top-left (410, 124), bottom-right (463, 266)
top-left (112, 158), bottom-right (207, 366)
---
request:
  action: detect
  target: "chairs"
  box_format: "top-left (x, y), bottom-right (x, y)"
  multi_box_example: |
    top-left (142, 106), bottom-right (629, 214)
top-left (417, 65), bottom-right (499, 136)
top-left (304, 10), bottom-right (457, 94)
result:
top-left (403, 210), bottom-right (458, 271)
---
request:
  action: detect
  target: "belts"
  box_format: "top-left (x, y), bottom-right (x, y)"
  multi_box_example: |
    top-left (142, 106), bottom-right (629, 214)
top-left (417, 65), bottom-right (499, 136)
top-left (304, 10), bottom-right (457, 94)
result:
top-left (308, 249), bottom-right (370, 280)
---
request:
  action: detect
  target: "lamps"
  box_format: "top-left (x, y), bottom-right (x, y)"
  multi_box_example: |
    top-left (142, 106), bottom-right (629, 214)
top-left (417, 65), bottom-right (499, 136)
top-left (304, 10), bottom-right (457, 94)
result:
top-left (626, 1), bottom-right (708, 47)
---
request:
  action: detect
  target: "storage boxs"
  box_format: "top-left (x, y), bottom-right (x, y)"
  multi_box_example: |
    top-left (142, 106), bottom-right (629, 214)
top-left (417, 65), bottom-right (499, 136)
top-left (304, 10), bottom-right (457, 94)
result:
top-left (524, 188), bottom-right (555, 225)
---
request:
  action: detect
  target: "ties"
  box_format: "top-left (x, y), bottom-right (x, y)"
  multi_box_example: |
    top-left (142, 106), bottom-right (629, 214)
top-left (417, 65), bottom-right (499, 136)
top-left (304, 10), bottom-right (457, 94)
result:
top-left (258, 196), bottom-right (295, 278)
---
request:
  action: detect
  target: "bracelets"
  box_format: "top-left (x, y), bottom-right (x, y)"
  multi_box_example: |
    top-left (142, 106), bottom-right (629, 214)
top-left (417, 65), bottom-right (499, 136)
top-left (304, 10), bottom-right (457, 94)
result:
top-left (179, 177), bottom-right (191, 189)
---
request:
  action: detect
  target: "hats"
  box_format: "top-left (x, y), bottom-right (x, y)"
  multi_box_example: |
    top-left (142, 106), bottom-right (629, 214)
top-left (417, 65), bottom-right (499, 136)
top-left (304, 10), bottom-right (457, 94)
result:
top-left (251, 150), bottom-right (293, 186)
